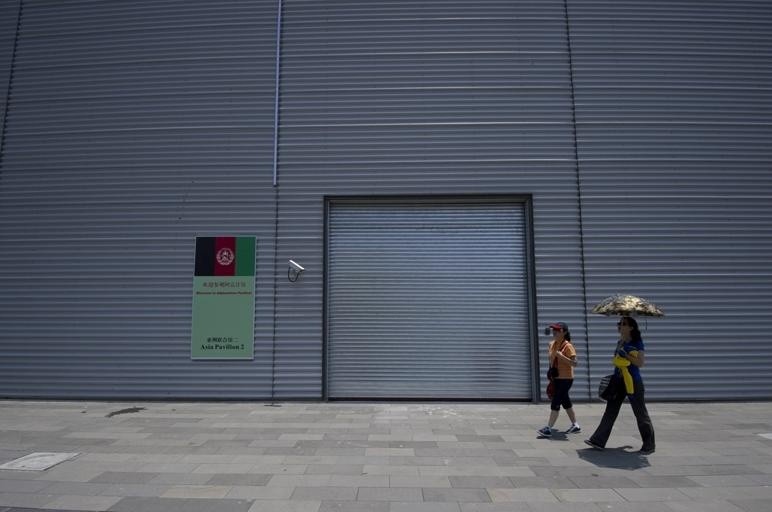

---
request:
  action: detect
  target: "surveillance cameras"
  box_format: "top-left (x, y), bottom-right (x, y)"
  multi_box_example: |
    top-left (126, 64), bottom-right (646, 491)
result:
top-left (288, 260), bottom-right (304, 273)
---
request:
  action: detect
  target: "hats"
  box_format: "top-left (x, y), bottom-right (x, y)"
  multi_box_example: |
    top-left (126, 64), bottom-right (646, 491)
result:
top-left (549, 321), bottom-right (568, 330)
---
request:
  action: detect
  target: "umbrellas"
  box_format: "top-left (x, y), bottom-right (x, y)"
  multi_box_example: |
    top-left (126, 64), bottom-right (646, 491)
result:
top-left (590, 292), bottom-right (666, 353)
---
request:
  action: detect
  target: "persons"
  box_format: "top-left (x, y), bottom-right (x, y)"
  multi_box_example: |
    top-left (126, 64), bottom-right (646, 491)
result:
top-left (535, 321), bottom-right (581, 438)
top-left (583, 314), bottom-right (656, 455)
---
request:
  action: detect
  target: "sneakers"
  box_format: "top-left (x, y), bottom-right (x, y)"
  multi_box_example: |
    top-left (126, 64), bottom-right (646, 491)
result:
top-left (566, 423), bottom-right (581, 434)
top-left (639, 446), bottom-right (655, 455)
top-left (536, 425), bottom-right (553, 437)
top-left (584, 438), bottom-right (605, 450)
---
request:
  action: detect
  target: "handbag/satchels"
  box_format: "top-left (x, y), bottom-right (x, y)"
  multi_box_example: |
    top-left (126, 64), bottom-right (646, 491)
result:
top-left (547, 367), bottom-right (559, 380)
top-left (598, 374), bottom-right (615, 403)
